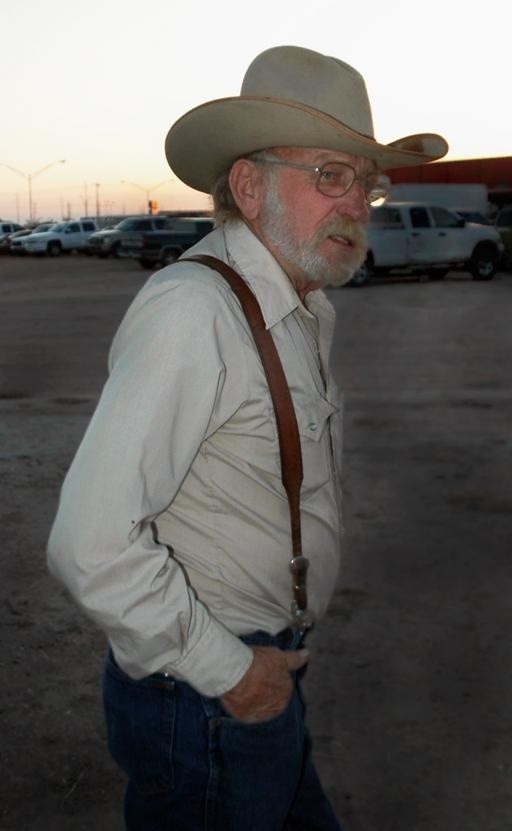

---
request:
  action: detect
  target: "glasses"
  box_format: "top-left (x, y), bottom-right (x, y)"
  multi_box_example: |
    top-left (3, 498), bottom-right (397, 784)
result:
top-left (246, 159), bottom-right (392, 208)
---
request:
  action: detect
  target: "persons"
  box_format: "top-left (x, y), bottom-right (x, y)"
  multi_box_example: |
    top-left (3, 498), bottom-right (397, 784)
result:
top-left (46, 46), bottom-right (448, 831)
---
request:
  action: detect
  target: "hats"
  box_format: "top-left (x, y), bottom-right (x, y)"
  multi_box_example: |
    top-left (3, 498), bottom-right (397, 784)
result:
top-left (165, 46), bottom-right (449, 194)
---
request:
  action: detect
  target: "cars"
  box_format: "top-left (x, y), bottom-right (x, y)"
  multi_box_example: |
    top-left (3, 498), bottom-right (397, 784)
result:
top-left (339, 201), bottom-right (505, 288)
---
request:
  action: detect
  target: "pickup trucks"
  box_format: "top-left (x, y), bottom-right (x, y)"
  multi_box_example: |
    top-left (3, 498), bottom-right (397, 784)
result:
top-left (1, 214), bottom-right (216, 272)
